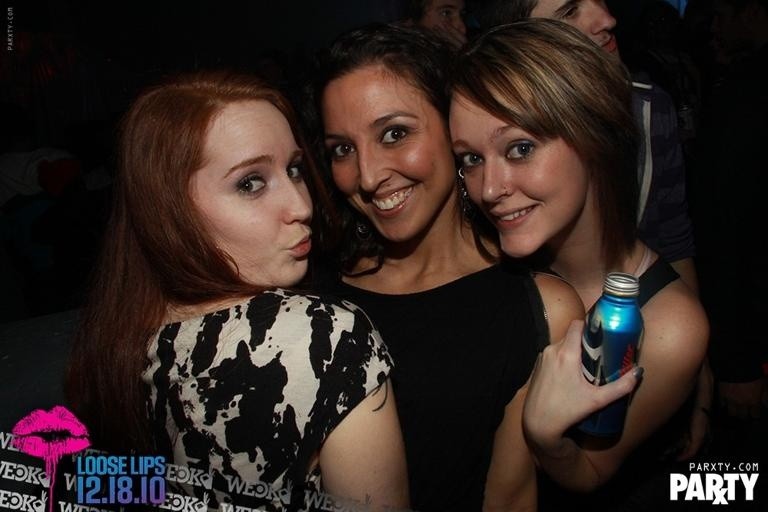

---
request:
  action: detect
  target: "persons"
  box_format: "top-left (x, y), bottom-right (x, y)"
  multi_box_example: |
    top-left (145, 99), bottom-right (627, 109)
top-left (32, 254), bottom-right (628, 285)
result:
top-left (291, 22), bottom-right (588, 511)
top-left (59, 60), bottom-right (415, 511)
top-left (400, 2), bottom-right (768, 512)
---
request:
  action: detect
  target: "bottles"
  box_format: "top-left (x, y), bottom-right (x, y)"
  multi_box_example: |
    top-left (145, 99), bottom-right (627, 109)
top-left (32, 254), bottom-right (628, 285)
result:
top-left (564, 272), bottom-right (646, 448)
